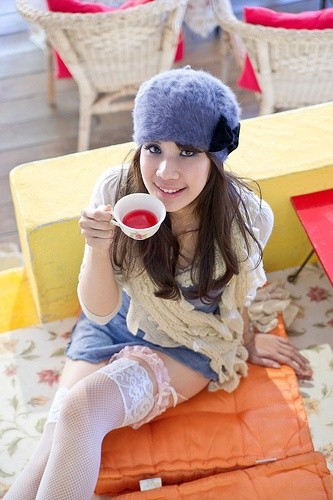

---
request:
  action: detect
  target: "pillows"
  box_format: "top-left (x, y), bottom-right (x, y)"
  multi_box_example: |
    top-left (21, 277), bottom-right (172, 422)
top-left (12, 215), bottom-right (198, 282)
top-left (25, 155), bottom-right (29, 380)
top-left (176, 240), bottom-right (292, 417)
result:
top-left (237, 5), bottom-right (333, 93)
top-left (46, 1), bottom-right (185, 81)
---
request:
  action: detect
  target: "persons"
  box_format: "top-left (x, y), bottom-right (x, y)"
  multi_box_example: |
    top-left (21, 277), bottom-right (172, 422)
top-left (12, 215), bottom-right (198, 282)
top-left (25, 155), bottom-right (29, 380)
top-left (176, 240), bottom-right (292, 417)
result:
top-left (2, 65), bottom-right (309, 500)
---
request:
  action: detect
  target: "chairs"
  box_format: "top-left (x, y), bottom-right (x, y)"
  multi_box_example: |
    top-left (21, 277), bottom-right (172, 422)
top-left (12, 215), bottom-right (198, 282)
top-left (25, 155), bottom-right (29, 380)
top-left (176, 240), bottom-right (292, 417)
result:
top-left (208, 0), bottom-right (332, 117)
top-left (15, 1), bottom-right (186, 154)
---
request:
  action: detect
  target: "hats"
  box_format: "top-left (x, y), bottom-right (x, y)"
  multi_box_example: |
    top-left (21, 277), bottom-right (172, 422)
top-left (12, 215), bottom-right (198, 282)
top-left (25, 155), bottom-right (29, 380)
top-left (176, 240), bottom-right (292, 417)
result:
top-left (131, 68), bottom-right (242, 164)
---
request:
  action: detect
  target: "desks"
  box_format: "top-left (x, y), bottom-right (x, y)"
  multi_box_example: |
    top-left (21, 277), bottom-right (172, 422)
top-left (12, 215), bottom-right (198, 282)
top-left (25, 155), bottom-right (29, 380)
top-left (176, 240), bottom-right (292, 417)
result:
top-left (288, 186), bottom-right (333, 285)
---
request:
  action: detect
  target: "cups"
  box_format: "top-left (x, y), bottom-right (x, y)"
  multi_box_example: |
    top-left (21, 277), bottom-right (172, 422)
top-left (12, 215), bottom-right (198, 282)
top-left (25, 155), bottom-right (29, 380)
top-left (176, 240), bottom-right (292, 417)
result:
top-left (104, 193), bottom-right (167, 240)
